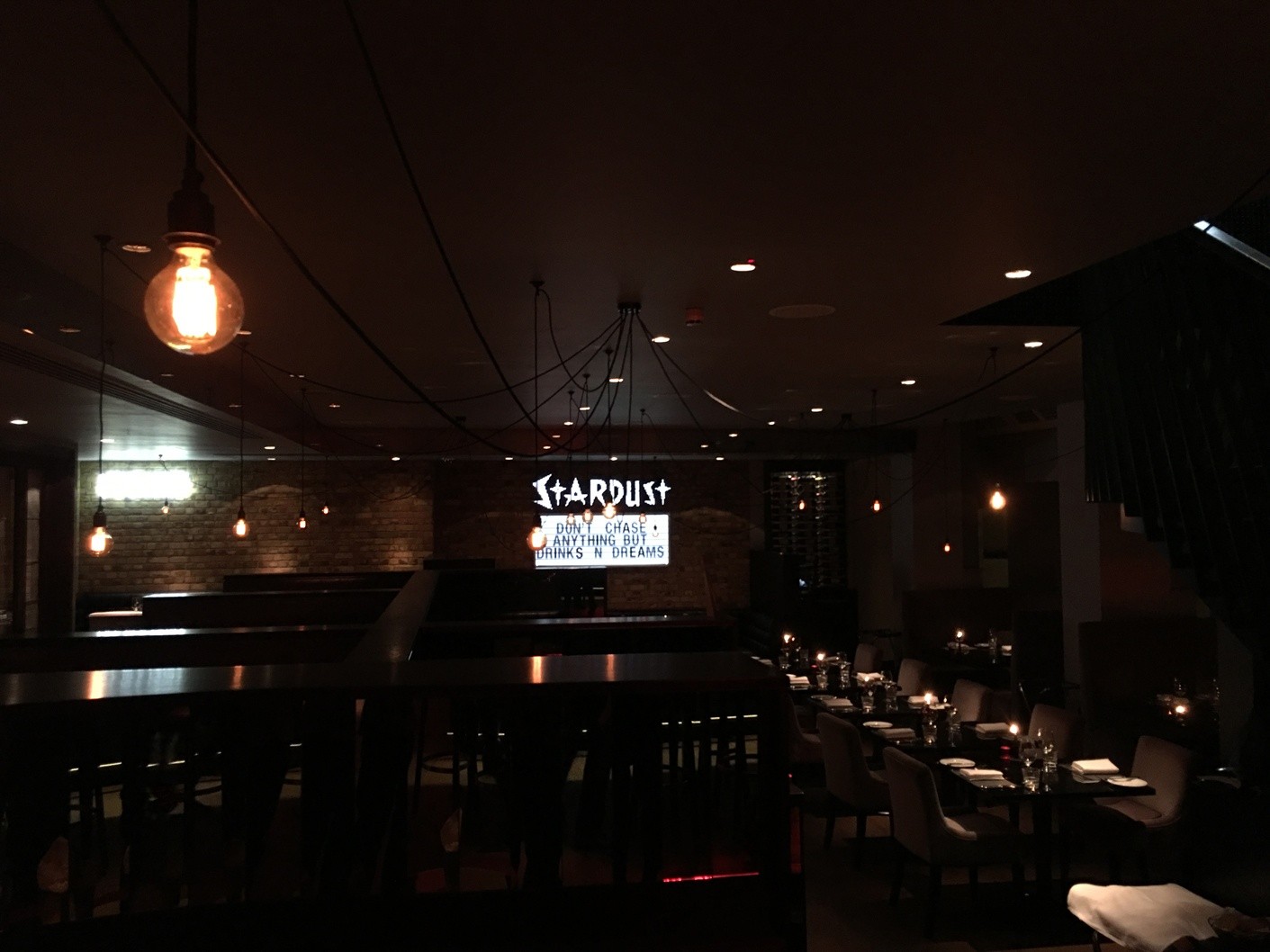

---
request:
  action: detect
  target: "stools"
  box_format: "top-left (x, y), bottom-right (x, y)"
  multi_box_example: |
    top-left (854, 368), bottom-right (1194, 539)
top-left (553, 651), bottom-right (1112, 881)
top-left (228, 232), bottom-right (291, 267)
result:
top-left (57, 710), bottom-right (755, 923)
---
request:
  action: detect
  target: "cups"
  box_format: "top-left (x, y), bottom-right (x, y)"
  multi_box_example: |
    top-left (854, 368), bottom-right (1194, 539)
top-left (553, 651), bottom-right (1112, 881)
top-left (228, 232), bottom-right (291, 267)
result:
top-left (1041, 731), bottom-right (1060, 792)
top-left (989, 626), bottom-right (997, 654)
top-left (921, 702), bottom-right (961, 745)
top-left (1212, 676), bottom-right (1226, 704)
top-left (1022, 767), bottom-right (1040, 789)
top-left (1163, 701), bottom-right (1175, 720)
top-left (1173, 676), bottom-right (1187, 697)
top-left (779, 634), bottom-right (899, 712)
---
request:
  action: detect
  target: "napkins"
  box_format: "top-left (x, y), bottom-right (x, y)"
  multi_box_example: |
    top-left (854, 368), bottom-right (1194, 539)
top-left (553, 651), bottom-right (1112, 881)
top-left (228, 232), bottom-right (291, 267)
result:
top-left (975, 722), bottom-right (1009, 734)
top-left (960, 768), bottom-right (1005, 781)
top-left (789, 676), bottom-right (810, 684)
top-left (1071, 758), bottom-right (1120, 775)
top-left (878, 727), bottom-right (915, 740)
top-left (821, 698), bottom-right (853, 708)
top-left (907, 695), bottom-right (940, 705)
top-left (856, 672), bottom-right (886, 683)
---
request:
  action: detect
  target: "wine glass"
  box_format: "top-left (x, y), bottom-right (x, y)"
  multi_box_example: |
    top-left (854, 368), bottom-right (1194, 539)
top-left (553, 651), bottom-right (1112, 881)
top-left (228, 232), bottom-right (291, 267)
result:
top-left (131, 597), bottom-right (141, 612)
top-left (1019, 739), bottom-right (1037, 768)
top-left (955, 628), bottom-right (965, 651)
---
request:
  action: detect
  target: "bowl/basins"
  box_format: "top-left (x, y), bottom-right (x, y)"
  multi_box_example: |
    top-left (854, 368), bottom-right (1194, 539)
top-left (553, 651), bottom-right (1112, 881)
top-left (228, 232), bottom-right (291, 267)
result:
top-left (1208, 915), bottom-right (1270, 942)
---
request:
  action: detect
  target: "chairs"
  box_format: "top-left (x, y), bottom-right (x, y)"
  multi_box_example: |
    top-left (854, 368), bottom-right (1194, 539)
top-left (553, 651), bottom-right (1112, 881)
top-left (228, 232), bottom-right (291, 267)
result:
top-left (853, 643), bottom-right (885, 673)
top-left (881, 746), bottom-right (1036, 941)
top-left (1059, 734), bottom-right (1199, 903)
top-left (951, 678), bottom-right (995, 723)
top-left (814, 711), bottom-right (897, 873)
top-left (789, 697), bottom-right (850, 812)
top-left (1009, 700), bottom-right (1087, 832)
top-left (895, 658), bottom-right (931, 696)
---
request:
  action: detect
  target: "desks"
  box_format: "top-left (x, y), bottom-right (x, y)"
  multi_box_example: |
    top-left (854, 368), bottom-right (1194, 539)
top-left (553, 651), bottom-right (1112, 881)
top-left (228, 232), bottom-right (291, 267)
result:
top-left (767, 669), bottom-right (1157, 952)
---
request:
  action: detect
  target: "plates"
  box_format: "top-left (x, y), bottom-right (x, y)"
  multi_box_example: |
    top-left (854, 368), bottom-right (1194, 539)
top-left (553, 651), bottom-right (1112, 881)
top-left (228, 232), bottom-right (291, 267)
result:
top-left (862, 721), bottom-right (893, 728)
top-left (939, 758), bottom-right (975, 768)
top-left (1103, 777), bottom-right (1148, 788)
top-left (811, 695), bottom-right (836, 700)
